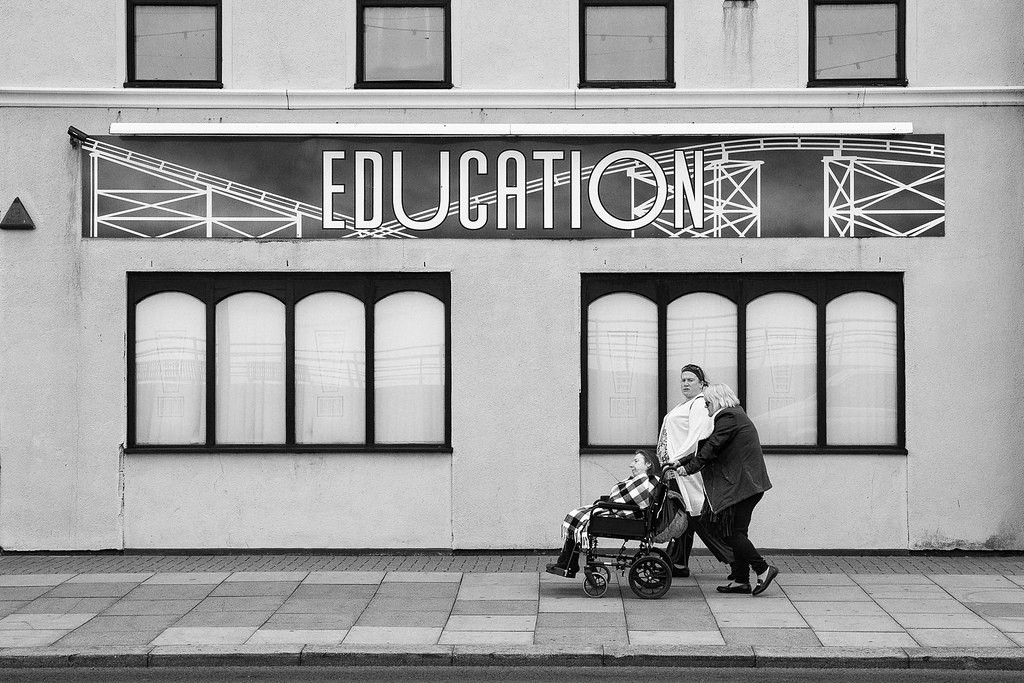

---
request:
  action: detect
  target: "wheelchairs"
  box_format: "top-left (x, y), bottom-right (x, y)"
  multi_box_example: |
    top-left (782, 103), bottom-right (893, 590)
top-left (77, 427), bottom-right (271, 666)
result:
top-left (545, 462), bottom-right (687, 600)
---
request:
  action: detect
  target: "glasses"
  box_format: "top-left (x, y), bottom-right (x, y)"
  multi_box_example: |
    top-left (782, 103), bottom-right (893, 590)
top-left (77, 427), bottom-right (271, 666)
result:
top-left (705, 401), bottom-right (710, 406)
top-left (681, 366), bottom-right (703, 380)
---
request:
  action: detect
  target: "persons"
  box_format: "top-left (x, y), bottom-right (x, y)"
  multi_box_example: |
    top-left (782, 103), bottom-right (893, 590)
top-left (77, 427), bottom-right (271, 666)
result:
top-left (673, 384), bottom-right (779, 596)
top-left (546, 449), bottom-right (663, 573)
top-left (656, 364), bottom-right (737, 581)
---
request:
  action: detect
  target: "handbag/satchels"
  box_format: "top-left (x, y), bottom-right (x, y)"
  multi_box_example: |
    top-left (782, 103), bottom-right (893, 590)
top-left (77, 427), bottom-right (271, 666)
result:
top-left (653, 490), bottom-right (689, 543)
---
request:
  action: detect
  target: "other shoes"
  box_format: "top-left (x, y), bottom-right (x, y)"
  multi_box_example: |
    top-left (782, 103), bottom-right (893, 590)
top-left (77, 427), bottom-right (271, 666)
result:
top-left (717, 583), bottom-right (751, 594)
top-left (547, 560), bottom-right (579, 573)
top-left (753, 565), bottom-right (779, 595)
top-left (727, 562), bottom-right (738, 580)
top-left (672, 564), bottom-right (690, 577)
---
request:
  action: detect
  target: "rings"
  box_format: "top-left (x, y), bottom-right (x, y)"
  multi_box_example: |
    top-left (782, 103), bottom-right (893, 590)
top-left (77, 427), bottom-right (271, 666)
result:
top-left (683, 473), bottom-right (684, 474)
